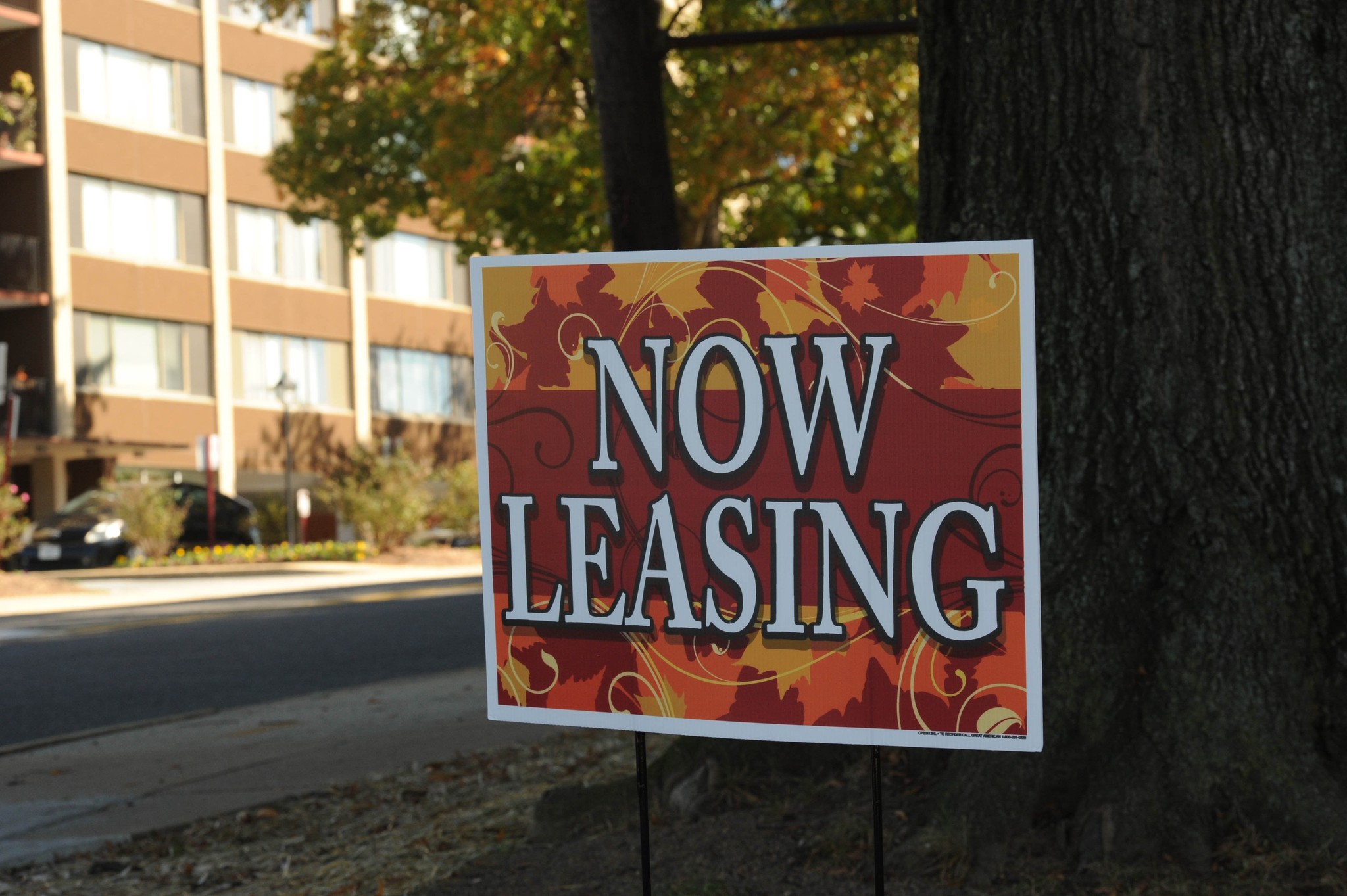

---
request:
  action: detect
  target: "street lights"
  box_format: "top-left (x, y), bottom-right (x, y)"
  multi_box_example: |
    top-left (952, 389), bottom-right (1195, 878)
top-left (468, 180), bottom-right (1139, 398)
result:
top-left (271, 371), bottom-right (301, 550)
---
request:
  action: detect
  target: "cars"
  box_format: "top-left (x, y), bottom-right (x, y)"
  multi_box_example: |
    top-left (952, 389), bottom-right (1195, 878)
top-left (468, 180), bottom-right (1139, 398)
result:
top-left (26, 478), bottom-right (263, 566)
